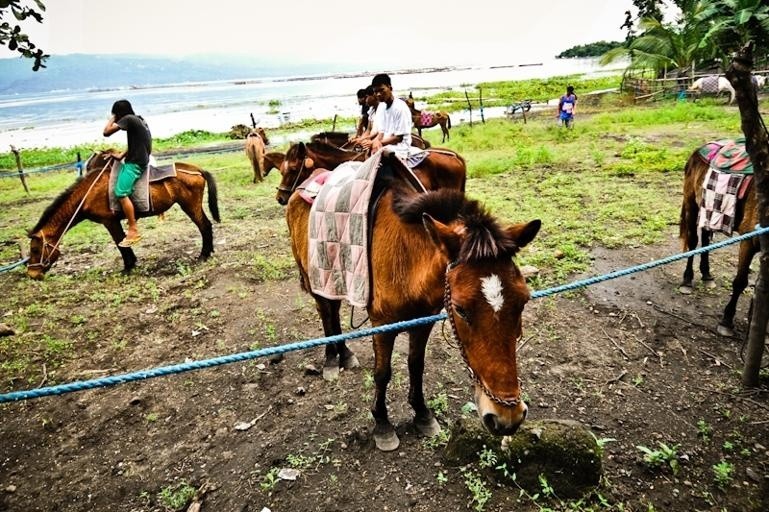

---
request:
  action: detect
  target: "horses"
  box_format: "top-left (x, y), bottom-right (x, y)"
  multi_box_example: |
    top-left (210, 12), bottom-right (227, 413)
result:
top-left (679, 148), bottom-right (761, 337)
top-left (688, 74), bottom-right (767, 105)
top-left (261, 130), bottom-right (467, 207)
top-left (25, 161), bottom-right (222, 280)
top-left (405, 92), bottom-right (451, 143)
top-left (285, 166), bottom-right (542, 453)
top-left (246, 127), bottom-right (269, 184)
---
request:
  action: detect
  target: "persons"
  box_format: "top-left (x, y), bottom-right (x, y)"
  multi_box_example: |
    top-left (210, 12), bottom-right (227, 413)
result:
top-left (359, 74), bottom-right (412, 156)
top-left (103, 99), bottom-right (151, 248)
top-left (352, 86), bottom-right (376, 144)
top-left (555, 86), bottom-right (578, 128)
top-left (356, 89), bottom-right (366, 139)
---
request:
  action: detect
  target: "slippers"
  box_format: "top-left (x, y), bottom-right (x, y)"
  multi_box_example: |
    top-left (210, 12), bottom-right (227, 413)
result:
top-left (118, 230), bottom-right (142, 248)
